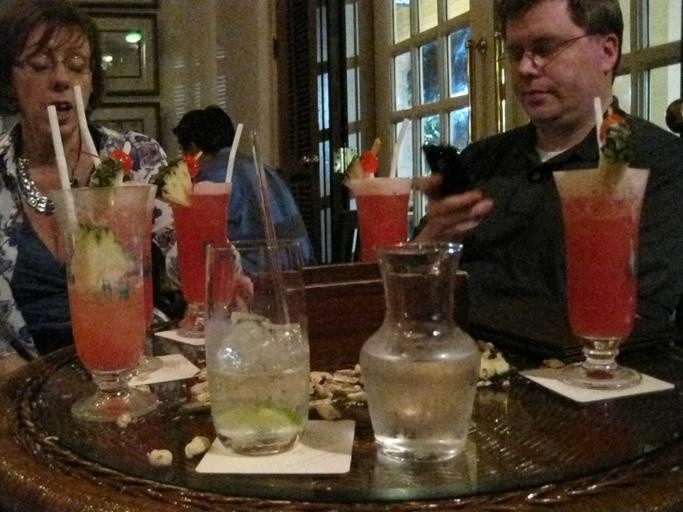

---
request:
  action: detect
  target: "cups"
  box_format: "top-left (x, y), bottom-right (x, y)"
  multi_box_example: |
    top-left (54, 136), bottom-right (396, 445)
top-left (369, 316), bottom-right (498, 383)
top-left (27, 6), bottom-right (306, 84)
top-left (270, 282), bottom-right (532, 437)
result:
top-left (198, 235), bottom-right (314, 456)
top-left (347, 174), bottom-right (412, 262)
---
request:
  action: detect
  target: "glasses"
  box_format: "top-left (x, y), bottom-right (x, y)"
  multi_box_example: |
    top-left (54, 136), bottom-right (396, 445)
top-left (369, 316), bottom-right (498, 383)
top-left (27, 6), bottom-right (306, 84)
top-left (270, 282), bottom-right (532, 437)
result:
top-left (506, 33), bottom-right (600, 60)
top-left (3, 53), bottom-right (100, 80)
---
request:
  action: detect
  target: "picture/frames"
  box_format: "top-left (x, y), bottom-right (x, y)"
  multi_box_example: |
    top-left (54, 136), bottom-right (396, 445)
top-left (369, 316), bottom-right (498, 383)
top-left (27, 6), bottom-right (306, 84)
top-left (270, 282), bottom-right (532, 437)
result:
top-left (83, 11), bottom-right (162, 145)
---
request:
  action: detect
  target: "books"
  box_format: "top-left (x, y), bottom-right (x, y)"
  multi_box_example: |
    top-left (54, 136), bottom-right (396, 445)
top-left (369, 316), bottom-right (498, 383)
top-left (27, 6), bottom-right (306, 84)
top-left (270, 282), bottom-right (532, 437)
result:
top-left (454, 306), bottom-right (679, 366)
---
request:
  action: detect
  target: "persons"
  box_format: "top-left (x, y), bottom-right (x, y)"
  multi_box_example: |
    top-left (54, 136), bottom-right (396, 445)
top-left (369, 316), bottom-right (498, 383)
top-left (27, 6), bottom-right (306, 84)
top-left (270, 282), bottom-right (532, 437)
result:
top-left (0, 2), bottom-right (256, 372)
top-left (172, 104), bottom-right (314, 268)
top-left (408, 1), bottom-right (682, 324)
top-left (158, 157), bottom-right (192, 205)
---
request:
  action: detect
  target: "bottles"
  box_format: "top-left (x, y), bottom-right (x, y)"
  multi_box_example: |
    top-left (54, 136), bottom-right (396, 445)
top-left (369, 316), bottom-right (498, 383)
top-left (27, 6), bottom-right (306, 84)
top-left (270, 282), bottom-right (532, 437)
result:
top-left (359, 236), bottom-right (478, 464)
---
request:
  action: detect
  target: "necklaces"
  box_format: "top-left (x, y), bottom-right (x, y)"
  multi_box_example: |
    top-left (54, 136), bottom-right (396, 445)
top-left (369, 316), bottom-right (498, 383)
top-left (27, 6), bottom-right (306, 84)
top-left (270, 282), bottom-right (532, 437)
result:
top-left (15, 130), bottom-right (84, 214)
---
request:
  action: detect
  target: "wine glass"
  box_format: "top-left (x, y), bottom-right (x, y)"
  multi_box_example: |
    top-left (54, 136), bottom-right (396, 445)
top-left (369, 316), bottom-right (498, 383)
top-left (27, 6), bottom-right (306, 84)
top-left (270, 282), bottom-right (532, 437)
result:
top-left (165, 180), bottom-right (235, 339)
top-left (53, 180), bottom-right (162, 421)
top-left (549, 164), bottom-right (648, 395)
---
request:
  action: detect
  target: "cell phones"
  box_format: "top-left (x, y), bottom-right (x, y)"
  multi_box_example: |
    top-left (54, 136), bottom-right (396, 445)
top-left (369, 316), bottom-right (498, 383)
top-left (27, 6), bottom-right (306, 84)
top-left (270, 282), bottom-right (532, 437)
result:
top-left (421, 144), bottom-right (474, 196)
